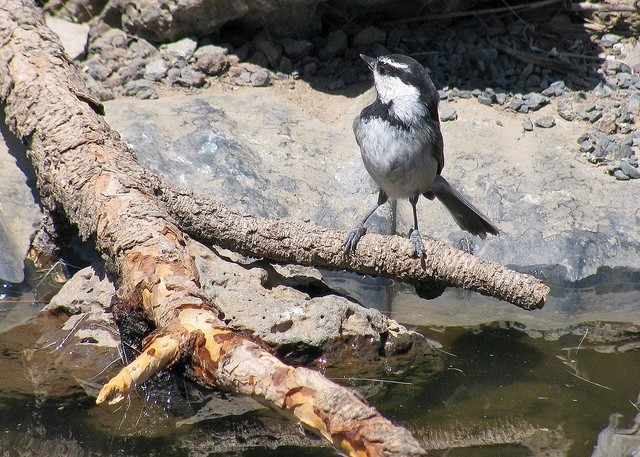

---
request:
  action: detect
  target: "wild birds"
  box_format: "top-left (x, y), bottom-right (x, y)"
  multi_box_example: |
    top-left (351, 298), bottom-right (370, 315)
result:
top-left (340, 54), bottom-right (500, 271)
